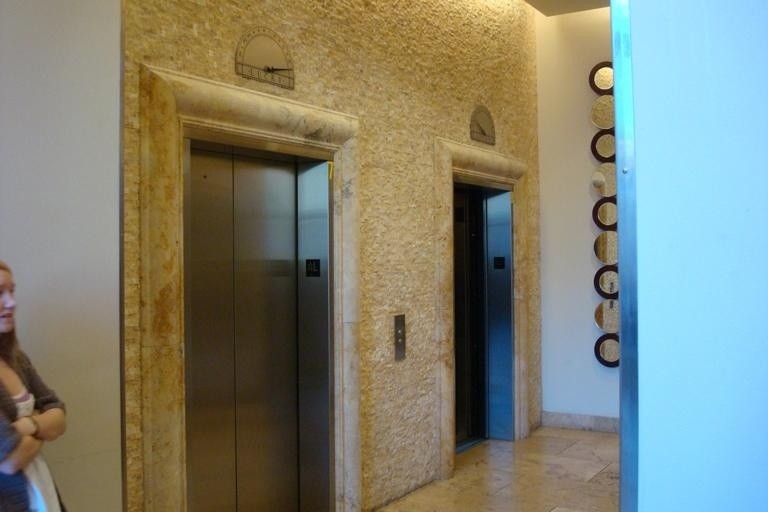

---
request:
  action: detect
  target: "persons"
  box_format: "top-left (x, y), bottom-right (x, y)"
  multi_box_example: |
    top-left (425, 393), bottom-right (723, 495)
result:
top-left (0, 260), bottom-right (67, 512)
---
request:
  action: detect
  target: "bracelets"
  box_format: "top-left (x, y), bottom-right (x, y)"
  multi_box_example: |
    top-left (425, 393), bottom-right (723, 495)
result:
top-left (28, 416), bottom-right (39, 436)
